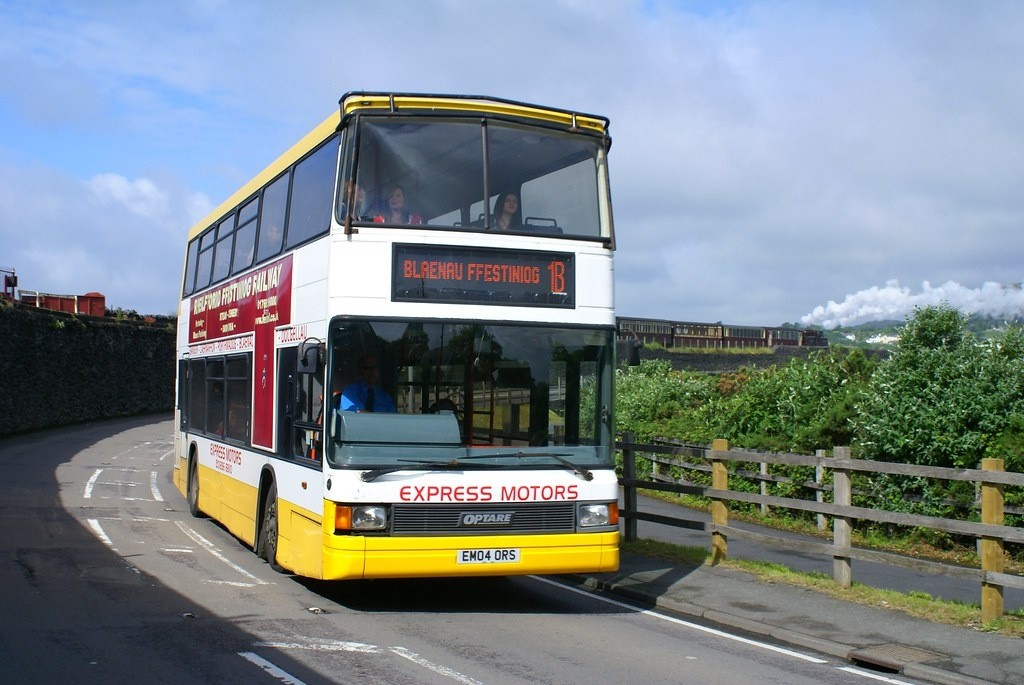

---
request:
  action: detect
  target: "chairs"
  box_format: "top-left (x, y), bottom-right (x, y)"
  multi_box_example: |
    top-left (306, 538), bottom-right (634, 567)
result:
top-left (453, 213), bottom-right (564, 232)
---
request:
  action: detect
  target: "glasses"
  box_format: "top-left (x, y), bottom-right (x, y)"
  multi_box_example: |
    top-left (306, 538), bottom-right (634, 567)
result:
top-left (359, 364), bottom-right (383, 369)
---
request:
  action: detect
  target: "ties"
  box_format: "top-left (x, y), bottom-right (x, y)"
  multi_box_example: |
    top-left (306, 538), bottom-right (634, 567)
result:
top-left (366, 385), bottom-right (374, 412)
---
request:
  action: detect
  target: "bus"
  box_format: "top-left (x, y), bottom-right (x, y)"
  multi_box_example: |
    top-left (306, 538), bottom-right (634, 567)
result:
top-left (172, 89), bottom-right (642, 580)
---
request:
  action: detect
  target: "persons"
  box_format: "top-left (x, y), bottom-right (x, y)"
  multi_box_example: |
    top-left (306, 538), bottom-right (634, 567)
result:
top-left (371, 184), bottom-right (427, 224)
top-left (340, 365), bottom-right (396, 414)
top-left (216, 396), bottom-right (251, 439)
top-left (246, 223), bottom-right (282, 266)
top-left (492, 190), bottom-right (519, 229)
top-left (430, 399), bottom-right (458, 419)
top-left (340, 180), bottom-right (372, 222)
